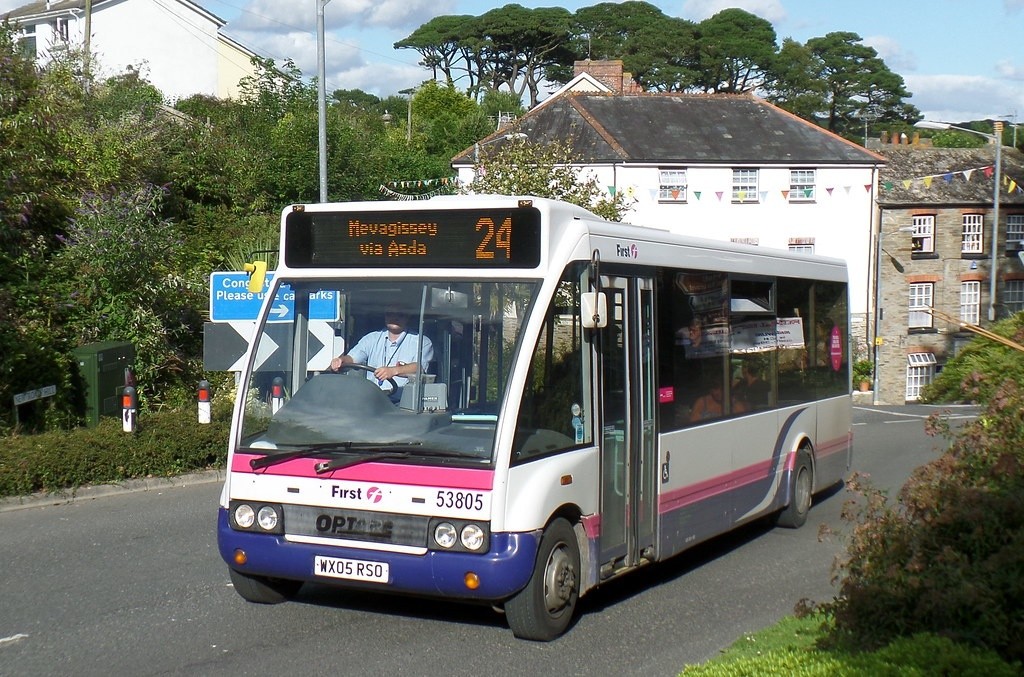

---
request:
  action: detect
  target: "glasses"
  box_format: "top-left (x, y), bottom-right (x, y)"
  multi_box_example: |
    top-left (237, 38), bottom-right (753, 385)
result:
top-left (385, 310), bottom-right (404, 317)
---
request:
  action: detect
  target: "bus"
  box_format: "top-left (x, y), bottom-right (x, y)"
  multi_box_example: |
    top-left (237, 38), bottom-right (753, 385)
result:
top-left (216, 195), bottom-right (853, 643)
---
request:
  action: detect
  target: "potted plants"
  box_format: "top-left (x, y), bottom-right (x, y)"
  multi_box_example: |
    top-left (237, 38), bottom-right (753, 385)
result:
top-left (856, 375), bottom-right (869, 392)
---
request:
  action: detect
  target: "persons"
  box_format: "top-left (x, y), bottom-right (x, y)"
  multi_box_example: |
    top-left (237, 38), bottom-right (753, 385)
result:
top-left (679, 315), bottom-right (774, 424)
top-left (331, 295), bottom-right (434, 404)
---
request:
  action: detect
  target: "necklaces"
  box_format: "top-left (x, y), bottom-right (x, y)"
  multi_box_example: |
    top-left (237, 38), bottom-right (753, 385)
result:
top-left (377, 329), bottom-right (409, 386)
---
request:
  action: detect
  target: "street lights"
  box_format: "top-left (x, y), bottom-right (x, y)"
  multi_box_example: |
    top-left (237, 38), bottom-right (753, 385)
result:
top-left (913, 122), bottom-right (1003, 321)
top-left (473, 133), bottom-right (528, 195)
top-left (872, 226), bottom-right (917, 406)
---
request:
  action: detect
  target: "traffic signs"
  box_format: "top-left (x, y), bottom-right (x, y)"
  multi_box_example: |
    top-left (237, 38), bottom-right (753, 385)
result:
top-left (209, 270), bottom-right (341, 323)
